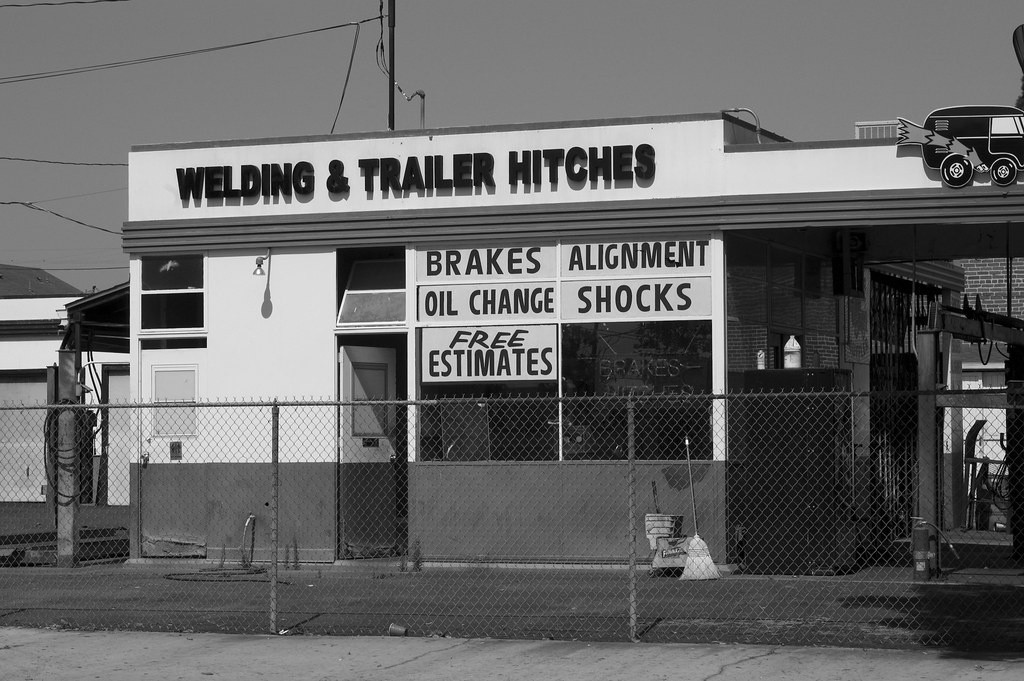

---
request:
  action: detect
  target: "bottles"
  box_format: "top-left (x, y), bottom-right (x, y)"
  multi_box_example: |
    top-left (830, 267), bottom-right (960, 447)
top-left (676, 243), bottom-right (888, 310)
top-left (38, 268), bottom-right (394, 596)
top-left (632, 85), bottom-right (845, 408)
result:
top-left (756, 350), bottom-right (765, 369)
top-left (783, 335), bottom-right (801, 369)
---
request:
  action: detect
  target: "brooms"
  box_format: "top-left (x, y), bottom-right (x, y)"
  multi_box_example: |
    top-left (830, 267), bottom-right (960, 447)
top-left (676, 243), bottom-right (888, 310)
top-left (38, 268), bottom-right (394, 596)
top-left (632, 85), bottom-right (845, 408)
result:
top-left (678, 436), bottom-right (721, 581)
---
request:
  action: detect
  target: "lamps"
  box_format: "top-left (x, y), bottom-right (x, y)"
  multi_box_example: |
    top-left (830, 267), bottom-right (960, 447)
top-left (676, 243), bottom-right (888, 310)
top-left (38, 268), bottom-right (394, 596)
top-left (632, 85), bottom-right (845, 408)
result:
top-left (253, 248), bottom-right (270, 276)
